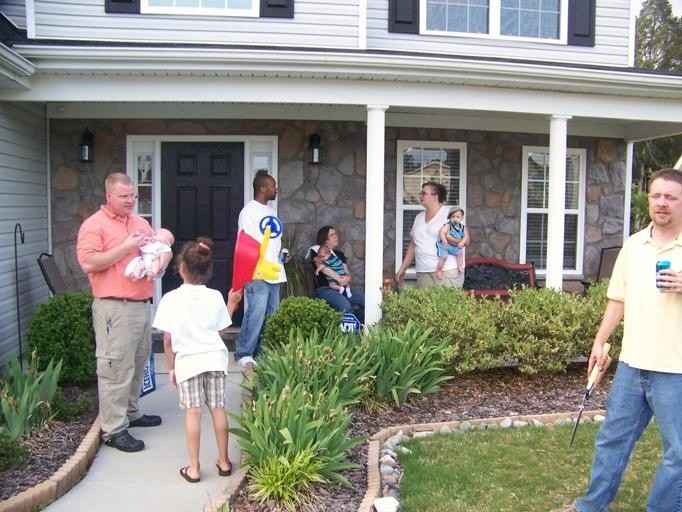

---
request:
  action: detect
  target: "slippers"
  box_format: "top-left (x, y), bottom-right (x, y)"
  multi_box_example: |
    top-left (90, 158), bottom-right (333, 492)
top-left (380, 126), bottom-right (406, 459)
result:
top-left (128, 415), bottom-right (161, 427)
top-left (216, 460), bottom-right (232, 477)
top-left (180, 465), bottom-right (200, 483)
top-left (105, 430), bottom-right (145, 452)
top-left (236, 356), bottom-right (257, 369)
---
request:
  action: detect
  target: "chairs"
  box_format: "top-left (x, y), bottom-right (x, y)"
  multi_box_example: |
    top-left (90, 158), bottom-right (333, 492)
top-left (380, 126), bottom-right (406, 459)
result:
top-left (464, 256), bottom-right (536, 299)
top-left (35, 252), bottom-right (68, 295)
top-left (579, 245), bottom-right (622, 288)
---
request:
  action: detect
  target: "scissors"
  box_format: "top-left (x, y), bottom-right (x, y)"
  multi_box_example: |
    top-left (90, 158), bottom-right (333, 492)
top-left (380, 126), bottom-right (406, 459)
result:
top-left (569, 342), bottom-right (613, 450)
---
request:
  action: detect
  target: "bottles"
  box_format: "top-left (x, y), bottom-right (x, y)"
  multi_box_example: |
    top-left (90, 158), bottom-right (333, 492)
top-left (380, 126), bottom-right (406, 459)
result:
top-left (279, 248), bottom-right (289, 263)
top-left (655, 262), bottom-right (671, 294)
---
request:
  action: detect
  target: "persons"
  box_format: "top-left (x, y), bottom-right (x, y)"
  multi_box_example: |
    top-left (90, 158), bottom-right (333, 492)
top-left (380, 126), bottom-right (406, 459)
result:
top-left (233, 170), bottom-right (292, 370)
top-left (75, 173), bottom-right (173, 451)
top-left (316, 246), bottom-right (352, 297)
top-left (304, 227), bottom-right (364, 322)
top-left (436, 206), bottom-right (468, 278)
top-left (152, 236), bottom-right (243, 483)
top-left (397, 182), bottom-right (466, 287)
top-left (571, 168), bottom-right (682, 508)
top-left (124, 228), bottom-right (174, 282)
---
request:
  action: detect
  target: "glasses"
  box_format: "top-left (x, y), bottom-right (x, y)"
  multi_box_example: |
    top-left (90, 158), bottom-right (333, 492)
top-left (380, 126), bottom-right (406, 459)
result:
top-left (108, 192), bottom-right (138, 204)
top-left (420, 191), bottom-right (437, 195)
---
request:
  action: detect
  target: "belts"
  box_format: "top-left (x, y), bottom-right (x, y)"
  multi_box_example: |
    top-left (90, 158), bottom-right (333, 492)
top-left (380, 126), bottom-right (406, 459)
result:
top-left (101, 296), bottom-right (151, 303)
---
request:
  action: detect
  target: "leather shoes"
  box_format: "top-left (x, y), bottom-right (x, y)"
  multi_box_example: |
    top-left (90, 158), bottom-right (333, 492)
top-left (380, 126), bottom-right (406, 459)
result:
top-left (549, 504), bottom-right (577, 512)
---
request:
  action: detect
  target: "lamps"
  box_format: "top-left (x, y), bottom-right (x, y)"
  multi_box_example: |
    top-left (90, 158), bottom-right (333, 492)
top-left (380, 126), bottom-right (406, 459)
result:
top-left (79, 125), bottom-right (95, 165)
top-left (308, 129), bottom-right (323, 166)
